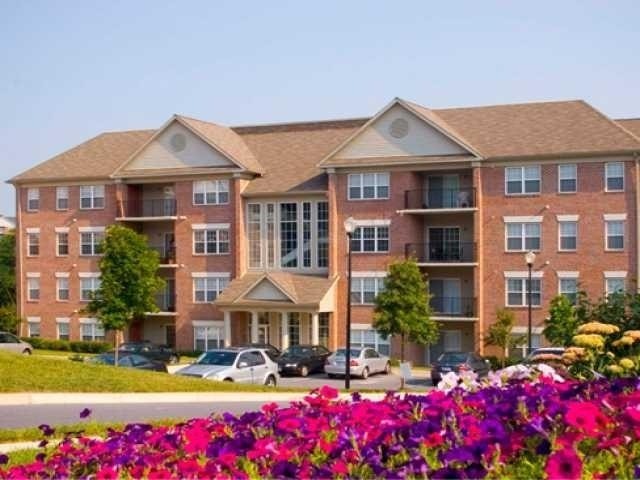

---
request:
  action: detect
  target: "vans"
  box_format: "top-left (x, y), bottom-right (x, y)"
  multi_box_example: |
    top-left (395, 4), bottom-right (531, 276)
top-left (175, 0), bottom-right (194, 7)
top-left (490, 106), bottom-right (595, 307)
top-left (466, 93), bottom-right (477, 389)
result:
top-left (0, 330), bottom-right (34, 356)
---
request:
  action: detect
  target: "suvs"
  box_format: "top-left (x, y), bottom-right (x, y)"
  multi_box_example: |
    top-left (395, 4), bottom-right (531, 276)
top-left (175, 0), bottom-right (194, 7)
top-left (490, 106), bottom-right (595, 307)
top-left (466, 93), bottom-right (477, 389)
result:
top-left (172, 345), bottom-right (281, 389)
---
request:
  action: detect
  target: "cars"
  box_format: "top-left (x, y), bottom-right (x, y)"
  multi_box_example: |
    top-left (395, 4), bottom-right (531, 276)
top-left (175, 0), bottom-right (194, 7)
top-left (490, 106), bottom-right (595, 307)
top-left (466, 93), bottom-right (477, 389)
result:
top-left (526, 346), bottom-right (571, 362)
top-left (430, 349), bottom-right (491, 387)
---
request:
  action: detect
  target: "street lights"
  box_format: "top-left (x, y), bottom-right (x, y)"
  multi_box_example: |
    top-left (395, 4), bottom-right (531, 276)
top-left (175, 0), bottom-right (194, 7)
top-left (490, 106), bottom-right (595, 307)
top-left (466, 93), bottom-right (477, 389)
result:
top-left (340, 212), bottom-right (360, 391)
top-left (523, 247), bottom-right (538, 355)
